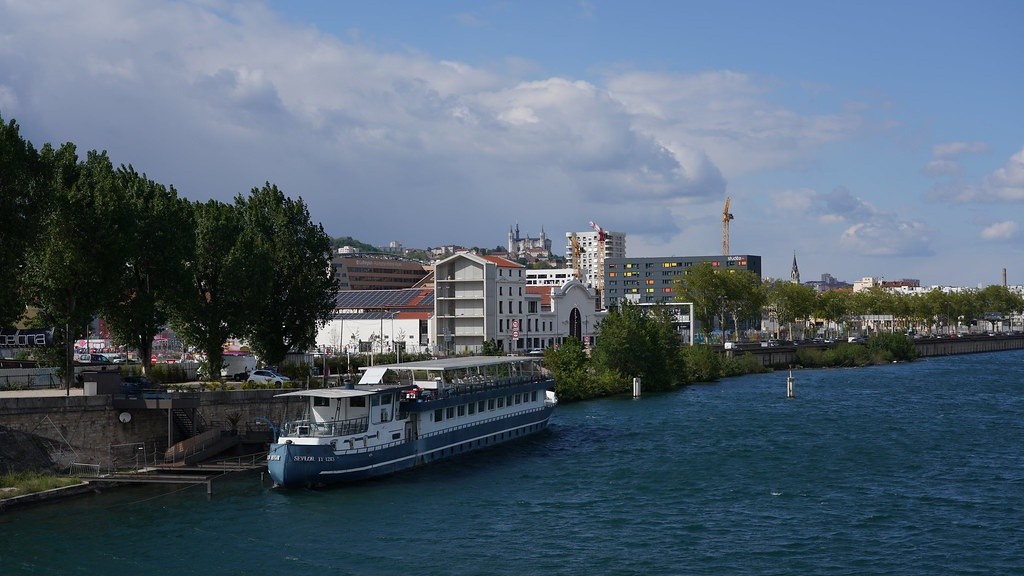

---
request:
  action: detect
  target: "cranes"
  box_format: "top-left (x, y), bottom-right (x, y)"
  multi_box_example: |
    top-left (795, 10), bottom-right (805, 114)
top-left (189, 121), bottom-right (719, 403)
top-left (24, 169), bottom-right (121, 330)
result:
top-left (588, 221), bottom-right (608, 292)
top-left (570, 234), bottom-right (586, 280)
top-left (721, 195), bottom-right (734, 257)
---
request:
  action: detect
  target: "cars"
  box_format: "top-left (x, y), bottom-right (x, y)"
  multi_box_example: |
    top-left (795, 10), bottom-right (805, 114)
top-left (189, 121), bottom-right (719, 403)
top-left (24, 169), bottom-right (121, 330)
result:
top-left (530, 348), bottom-right (546, 354)
top-left (70, 343), bottom-right (133, 363)
top-left (767, 331), bottom-right (1014, 346)
top-left (247, 369), bottom-right (292, 388)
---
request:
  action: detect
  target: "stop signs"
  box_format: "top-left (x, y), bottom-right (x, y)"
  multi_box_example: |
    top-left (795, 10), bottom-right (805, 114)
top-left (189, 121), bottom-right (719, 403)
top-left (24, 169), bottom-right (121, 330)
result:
top-left (151, 355), bottom-right (156, 364)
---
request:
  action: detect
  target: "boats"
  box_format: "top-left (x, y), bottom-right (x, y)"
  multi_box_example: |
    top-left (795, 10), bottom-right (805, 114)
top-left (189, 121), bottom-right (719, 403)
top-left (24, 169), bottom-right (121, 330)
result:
top-left (266, 352), bottom-right (559, 489)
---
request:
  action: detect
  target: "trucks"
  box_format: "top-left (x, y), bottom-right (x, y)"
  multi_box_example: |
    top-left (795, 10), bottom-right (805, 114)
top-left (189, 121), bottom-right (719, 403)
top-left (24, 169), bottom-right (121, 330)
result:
top-left (195, 352), bottom-right (257, 383)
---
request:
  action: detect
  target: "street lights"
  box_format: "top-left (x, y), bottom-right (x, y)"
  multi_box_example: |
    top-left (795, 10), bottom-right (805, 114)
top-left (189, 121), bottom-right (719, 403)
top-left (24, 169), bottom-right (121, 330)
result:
top-left (718, 295), bottom-right (728, 349)
top-left (830, 298), bottom-right (839, 344)
top-left (944, 300), bottom-right (952, 335)
top-left (384, 310), bottom-right (401, 353)
